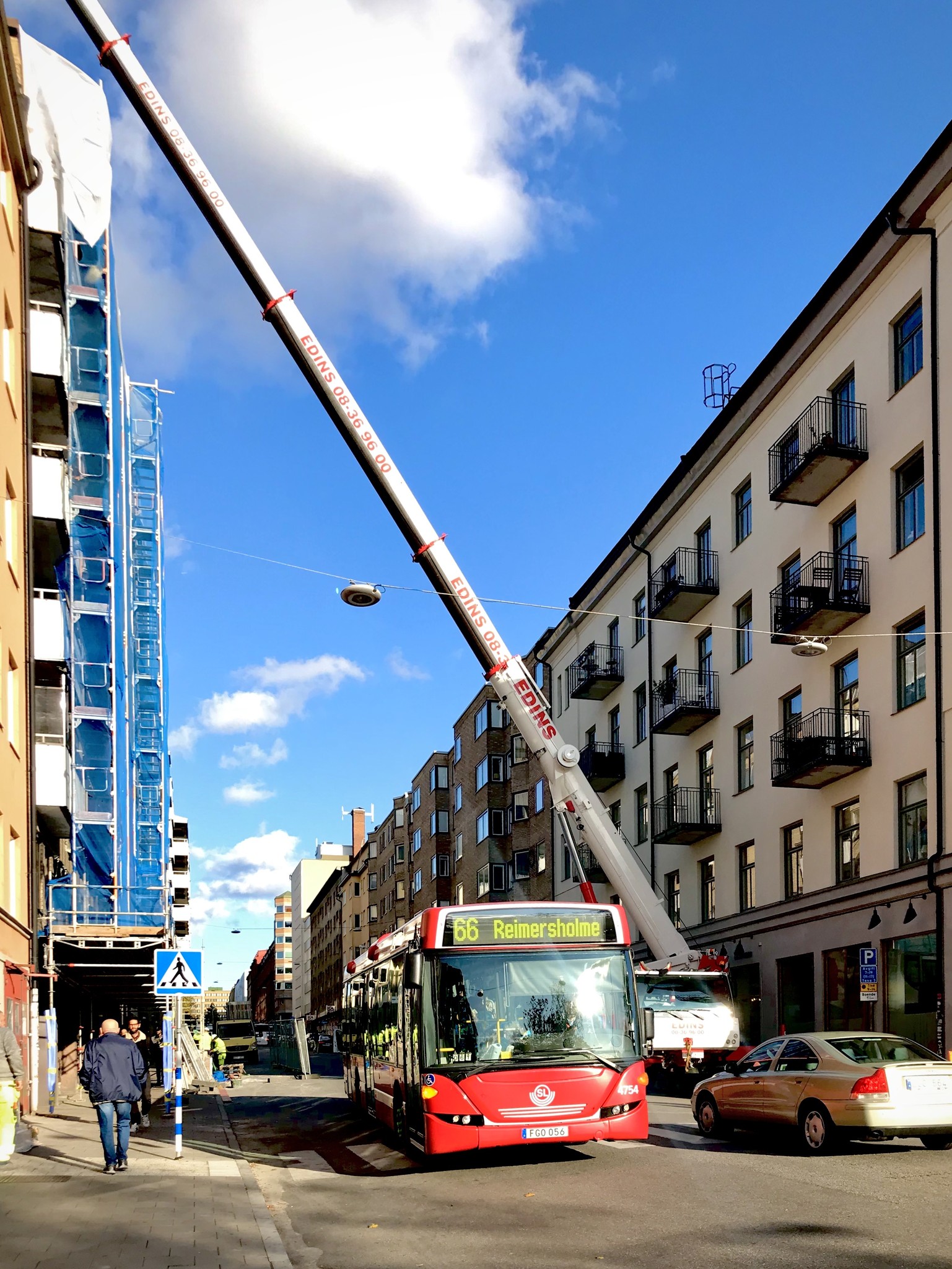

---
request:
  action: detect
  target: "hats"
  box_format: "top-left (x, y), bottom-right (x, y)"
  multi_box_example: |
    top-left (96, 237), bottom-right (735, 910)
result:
top-left (156, 1026), bottom-right (161, 1031)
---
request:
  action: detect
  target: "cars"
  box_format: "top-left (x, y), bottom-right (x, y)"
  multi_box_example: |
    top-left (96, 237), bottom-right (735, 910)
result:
top-left (690, 1031), bottom-right (952, 1155)
top-left (253, 1023), bottom-right (343, 1055)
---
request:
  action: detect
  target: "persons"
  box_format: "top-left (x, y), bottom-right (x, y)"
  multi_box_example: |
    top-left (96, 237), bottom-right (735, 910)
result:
top-left (313, 1030), bottom-right (319, 1053)
top-left (453, 978), bottom-right (518, 1024)
top-left (90, 1016), bottom-right (163, 1133)
top-left (210, 1034), bottom-right (227, 1066)
top-left (364, 1022), bottom-right (418, 1059)
top-left (0, 1010), bottom-right (24, 1161)
top-left (556, 996), bottom-right (593, 1035)
top-left (78, 1019), bottom-right (148, 1174)
top-left (193, 1027), bottom-right (212, 1054)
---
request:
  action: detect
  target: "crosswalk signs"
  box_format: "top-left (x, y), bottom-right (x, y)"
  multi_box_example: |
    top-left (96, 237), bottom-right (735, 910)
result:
top-left (154, 948), bottom-right (205, 997)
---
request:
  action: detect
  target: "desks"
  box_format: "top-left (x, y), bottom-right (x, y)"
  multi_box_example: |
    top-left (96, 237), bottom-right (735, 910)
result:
top-left (788, 586), bottom-right (831, 623)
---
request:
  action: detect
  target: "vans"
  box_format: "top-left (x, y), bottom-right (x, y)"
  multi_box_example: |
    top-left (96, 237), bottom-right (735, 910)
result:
top-left (213, 1018), bottom-right (263, 1065)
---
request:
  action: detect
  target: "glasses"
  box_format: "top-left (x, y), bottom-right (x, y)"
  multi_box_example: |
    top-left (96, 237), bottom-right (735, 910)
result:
top-left (129, 1024), bottom-right (138, 1027)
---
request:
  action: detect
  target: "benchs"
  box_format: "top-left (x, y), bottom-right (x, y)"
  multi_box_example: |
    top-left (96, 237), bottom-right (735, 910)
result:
top-left (841, 1048), bottom-right (910, 1060)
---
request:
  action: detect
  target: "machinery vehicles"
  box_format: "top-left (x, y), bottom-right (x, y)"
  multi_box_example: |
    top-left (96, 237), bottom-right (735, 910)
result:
top-left (59, 0), bottom-right (749, 1096)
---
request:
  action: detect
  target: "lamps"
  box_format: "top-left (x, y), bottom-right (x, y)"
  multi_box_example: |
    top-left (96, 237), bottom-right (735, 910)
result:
top-left (719, 939), bottom-right (736, 956)
top-left (791, 636), bottom-right (828, 657)
top-left (341, 580), bottom-right (382, 607)
top-left (903, 894), bottom-right (926, 924)
top-left (734, 935), bottom-right (754, 957)
top-left (868, 903), bottom-right (891, 930)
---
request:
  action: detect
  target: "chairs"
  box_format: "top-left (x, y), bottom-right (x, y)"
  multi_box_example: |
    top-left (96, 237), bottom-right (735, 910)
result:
top-left (773, 567), bottom-right (863, 633)
top-left (444, 996), bottom-right (494, 1036)
top-left (688, 685), bottom-right (709, 708)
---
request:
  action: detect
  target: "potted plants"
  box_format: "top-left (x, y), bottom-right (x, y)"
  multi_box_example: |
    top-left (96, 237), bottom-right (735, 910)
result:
top-left (648, 674), bottom-right (677, 715)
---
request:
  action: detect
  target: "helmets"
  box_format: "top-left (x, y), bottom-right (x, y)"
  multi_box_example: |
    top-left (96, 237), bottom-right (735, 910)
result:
top-left (204, 1027), bottom-right (209, 1031)
top-left (212, 1034), bottom-right (217, 1040)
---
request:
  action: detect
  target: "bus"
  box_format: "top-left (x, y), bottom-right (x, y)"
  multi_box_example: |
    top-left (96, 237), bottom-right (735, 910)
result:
top-left (344, 901), bottom-right (655, 1171)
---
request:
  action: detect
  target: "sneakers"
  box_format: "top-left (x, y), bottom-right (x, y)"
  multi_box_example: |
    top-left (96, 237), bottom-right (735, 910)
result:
top-left (117, 1159), bottom-right (129, 1169)
top-left (102, 1165), bottom-right (116, 1174)
top-left (130, 1123), bottom-right (139, 1133)
top-left (141, 1112), bottom-right (150, 1127)
